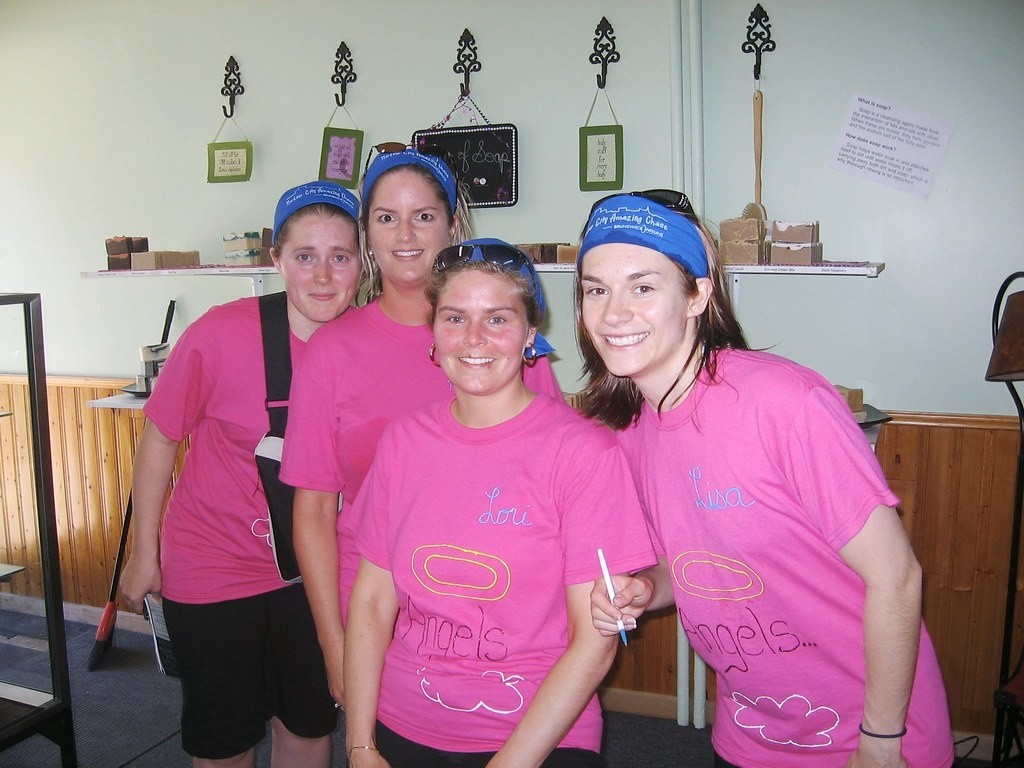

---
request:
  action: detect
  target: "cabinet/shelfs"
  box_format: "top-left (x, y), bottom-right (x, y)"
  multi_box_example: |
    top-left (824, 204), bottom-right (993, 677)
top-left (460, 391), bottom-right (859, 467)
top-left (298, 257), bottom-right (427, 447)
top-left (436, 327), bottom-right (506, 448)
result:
top-left (0, 293), bottom-right (79, 768)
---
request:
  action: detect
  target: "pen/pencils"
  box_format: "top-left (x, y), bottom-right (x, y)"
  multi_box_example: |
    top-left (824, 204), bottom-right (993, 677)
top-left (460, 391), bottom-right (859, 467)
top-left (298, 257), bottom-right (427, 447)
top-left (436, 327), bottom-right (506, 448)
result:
top-left (597, 548), bottom-right (628, 645)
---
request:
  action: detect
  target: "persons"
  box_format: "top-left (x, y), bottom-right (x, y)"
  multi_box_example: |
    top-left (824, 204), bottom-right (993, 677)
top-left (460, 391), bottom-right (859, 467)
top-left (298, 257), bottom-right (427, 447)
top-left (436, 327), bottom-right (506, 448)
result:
top-left (277, 152), bottom-right (573, 712)
top-left (118, 182), bottom-right (385, 768)
top-left (341, 238), bottom-right (659, 768)
top-left (573, 189), bottom-right (955, 768)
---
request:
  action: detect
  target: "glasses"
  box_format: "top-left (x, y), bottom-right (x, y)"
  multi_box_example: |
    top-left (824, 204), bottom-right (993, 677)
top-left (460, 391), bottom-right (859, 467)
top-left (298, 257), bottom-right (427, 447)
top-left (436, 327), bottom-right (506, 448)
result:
top-left (591, 188), bottom-right (701, 226)
top-left (363, 141), bottom-right (458, 188)
top-left (433, 244), bottom-right (536, 292)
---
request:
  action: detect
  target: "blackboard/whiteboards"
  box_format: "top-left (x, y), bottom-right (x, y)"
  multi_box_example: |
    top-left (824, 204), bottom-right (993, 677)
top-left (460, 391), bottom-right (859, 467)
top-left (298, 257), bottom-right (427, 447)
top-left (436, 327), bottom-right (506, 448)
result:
top-left (408, 122), bottom-right (518, 208)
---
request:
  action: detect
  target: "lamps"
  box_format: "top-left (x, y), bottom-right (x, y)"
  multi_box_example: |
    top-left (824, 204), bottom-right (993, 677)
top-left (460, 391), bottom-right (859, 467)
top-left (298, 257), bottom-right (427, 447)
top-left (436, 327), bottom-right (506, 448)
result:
top-left (984, 272), bottom-right (1024, 768)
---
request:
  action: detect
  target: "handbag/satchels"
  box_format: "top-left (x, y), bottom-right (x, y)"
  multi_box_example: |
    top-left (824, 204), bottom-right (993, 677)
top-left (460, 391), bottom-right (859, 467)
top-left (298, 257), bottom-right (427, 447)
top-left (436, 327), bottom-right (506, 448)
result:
top-left (255, 291), bottom-right (306, 583)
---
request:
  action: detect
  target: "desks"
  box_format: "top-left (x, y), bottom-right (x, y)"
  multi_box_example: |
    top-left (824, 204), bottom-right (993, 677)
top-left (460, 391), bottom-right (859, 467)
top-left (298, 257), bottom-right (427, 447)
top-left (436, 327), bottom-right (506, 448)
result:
top-left (88, 395), bottom-right (890, 730)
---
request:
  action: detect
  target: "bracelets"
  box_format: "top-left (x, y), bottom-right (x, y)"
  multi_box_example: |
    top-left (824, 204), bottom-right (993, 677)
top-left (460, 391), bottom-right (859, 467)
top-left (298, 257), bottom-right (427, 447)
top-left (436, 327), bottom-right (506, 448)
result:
top-left (344, 745), bottom-right (381, 762)
top-left (858, 723), bottom-right (907, 737)
